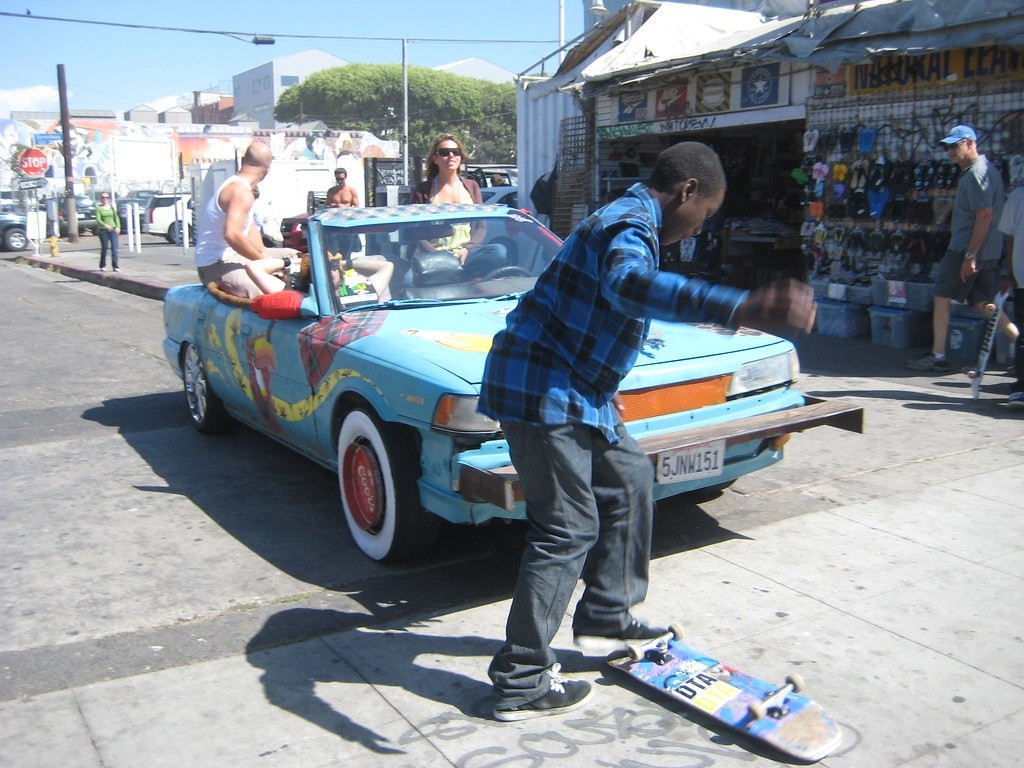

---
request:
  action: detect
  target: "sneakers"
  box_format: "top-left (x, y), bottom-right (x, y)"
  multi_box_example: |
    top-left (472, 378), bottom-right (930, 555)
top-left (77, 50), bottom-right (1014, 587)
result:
top-left (1007, 391), bottom-right (1024, 411)
top-left (573, 616), bottom-right (668, 658)
top-left (905, 351), bottom-right (951, 372)
top-left (492, 658), bottom-right (593, 720)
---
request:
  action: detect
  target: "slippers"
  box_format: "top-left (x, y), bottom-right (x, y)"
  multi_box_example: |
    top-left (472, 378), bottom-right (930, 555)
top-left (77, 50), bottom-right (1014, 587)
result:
top-left (796, 125), bottom-right (1024, 287)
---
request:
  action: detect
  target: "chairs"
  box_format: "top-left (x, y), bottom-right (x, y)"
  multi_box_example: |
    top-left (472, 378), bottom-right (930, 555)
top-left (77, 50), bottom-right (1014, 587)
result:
top-left (403, 250), bottom-right (472, 298)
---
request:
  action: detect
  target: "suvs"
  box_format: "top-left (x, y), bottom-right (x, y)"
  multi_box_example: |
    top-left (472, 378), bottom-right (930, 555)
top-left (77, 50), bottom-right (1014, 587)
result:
top-left (39, 195), bottom-right (99, 237)
top-left (142, 194), bottom-right (195, 246)
top-left (118, 198), bottom-right (148, 233)
top-left (468, 164), bottom-right (518, 186)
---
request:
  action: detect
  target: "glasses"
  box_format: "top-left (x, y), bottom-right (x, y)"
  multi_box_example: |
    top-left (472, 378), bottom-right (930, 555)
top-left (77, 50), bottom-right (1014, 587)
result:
top-left (336, 177), bottom-right (345, 180)
top-left (330, 262), bottom-right (339, 271)
top-left (101, 196), bottom-right (109, 199)
top-left (943, 139), bottom-right (969, 151)
top-left (434, 147), bottom-right (460, 158)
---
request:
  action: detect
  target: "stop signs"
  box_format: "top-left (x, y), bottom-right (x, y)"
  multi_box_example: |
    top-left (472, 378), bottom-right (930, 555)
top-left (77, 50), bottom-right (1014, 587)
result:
top-left (18, 148), bottom-right (48, 177)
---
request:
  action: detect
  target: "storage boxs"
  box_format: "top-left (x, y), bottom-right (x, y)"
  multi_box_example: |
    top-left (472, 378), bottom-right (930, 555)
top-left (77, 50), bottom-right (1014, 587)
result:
top-left (809, 272), bottom-right (1016, 365)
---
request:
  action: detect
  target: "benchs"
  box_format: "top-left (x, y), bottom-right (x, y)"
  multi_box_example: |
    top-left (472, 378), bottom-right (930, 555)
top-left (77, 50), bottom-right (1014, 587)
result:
top-left (345, 256), bottom-right (412, 294)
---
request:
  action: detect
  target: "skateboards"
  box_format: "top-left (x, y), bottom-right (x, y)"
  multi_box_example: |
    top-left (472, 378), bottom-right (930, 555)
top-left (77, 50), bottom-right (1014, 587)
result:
top-left (604, 623), bottom-right (841, 764)
top-left (965, 289), bottom-right (1010, 401)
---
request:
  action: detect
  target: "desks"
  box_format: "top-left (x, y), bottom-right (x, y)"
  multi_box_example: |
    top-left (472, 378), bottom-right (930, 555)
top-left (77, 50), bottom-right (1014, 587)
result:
top-left (719, 229), bottom-right (801, 283)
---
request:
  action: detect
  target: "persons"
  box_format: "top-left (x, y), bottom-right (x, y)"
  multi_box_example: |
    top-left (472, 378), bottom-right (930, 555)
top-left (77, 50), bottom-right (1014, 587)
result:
top-left (903, 125), bottom-right (1021, 376)
top-left (488, 142), bottom-right (816, 721)
top-left (996, 155), bottom-right (1024, 410)
top-left (196, 141), bottom-right (395, 311)
top-left (491, 174), bottom-right (513, 206)
top-left (407, 133), bottom-right (487, 267)
top-left (96, 192), bottom-right (123, 272)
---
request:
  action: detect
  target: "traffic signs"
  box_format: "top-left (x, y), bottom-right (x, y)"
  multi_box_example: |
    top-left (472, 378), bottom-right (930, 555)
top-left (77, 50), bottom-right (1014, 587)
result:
top-left (19, 177), bottom-right (48, 190)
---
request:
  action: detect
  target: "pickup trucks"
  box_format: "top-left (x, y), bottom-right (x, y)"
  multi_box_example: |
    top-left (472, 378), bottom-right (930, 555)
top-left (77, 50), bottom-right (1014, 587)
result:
top-left (161, 204), bottom-right (863, 561)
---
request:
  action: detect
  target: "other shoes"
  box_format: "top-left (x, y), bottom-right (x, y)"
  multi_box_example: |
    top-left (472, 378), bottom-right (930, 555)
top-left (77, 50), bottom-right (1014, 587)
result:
top-left (114, 268), bottom-right (120, 272)
top-left (101, 267), bottom-right (104, 271)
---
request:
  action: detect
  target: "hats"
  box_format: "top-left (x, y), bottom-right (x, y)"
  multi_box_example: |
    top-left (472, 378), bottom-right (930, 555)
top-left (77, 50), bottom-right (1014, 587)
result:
top-left (937, 125), bottom-right (977, 147)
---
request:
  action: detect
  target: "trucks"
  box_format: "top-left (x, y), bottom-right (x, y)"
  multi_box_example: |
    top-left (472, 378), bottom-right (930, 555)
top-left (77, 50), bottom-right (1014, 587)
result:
top-left (188, 162), bottom-right (367, 246)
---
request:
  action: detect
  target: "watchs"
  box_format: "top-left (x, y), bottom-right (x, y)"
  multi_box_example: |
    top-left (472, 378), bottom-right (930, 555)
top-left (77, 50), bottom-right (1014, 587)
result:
top-left (965, 252), bottom-right (976, 258)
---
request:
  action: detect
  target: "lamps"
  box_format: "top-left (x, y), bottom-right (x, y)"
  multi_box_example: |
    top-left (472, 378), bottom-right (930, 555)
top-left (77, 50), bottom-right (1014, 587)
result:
top-left (251, 36), bottom-right (276, 45)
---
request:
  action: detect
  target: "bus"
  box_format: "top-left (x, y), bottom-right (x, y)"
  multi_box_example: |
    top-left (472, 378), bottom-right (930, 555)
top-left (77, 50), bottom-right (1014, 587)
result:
top-left (125, 190), bottom-right (164, 199)
top-left (171, 129), bottom-right (399, 194)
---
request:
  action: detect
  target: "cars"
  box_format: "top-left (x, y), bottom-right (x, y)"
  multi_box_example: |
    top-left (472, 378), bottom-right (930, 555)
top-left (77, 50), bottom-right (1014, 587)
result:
top-left (0, 212), bottom-right (30, 251)
top-left (480, 187), bottom-right (520, 205)
top-left (0, 190), bottom-right (20, 212)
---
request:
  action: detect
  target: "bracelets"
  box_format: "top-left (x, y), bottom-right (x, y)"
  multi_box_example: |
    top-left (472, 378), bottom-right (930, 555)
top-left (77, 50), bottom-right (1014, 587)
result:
top-left (282, 258), bottom-right (291, 267)
top-left (346, 259), bottom-right (352, 269)
top-left (464, 247), bottom-right (470, 254)
